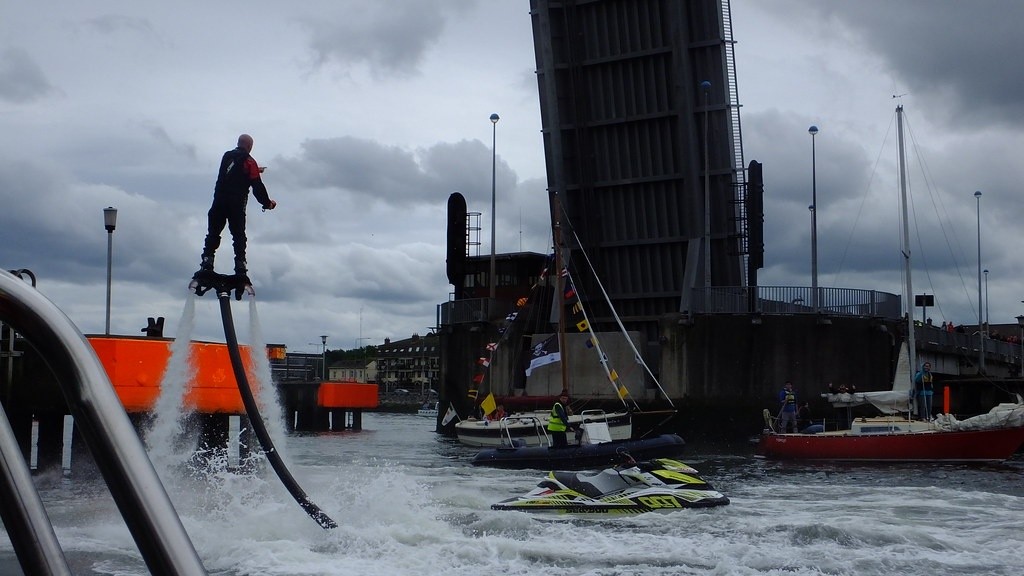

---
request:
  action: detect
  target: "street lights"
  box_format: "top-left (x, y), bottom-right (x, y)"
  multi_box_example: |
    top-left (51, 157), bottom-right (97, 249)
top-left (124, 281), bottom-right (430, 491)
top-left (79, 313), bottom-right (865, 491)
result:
top-left (488, 113), bottom-right (500, 319)
top-left (983, 269), bottom-right (990, 339)
top-left (103, 207), bottom-right (118, 335)
top-left (974, 191), bottom-right (986, 376)
top-left (320, 336), bottom-right (328, 381)
top-left (809, 126), bottom-right (818, 311)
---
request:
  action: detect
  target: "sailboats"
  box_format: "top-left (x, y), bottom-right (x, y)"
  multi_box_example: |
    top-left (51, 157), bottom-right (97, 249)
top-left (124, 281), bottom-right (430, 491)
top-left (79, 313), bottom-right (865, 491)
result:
top-left (760, 94), bottom-right (1024, 461)
top-left (455, 190), bottom-right (677, 443)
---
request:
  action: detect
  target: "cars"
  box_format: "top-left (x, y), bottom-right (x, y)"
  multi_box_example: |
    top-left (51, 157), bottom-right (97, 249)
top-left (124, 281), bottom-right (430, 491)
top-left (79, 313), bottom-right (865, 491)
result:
top-left (424, 389), bottom-right (437, 395)
top-left (393, 388), bottom-right (408, 394)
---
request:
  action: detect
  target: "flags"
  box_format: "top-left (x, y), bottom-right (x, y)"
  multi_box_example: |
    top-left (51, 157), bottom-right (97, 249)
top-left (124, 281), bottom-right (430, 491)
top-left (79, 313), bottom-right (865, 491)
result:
top-left (561, 266), bottom-right (628, 400)
top-left (442, 267), bottom-right (548, 427)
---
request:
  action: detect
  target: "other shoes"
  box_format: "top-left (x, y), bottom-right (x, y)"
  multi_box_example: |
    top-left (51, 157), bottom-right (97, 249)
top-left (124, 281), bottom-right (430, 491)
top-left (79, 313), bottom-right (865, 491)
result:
top-left (201, 264), bottom-right (213, 271)
top-left (236, 268), bottom-right (246, 275)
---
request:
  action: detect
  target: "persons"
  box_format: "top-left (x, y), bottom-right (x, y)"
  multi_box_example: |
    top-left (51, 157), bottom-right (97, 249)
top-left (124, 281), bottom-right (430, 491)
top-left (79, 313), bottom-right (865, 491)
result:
top-left (983, 322), bottom-right (1022, 345)
top-left (548, 391), bottom-right (573, 447)
top-left (202, 134), bottom-right (276, 276)
top-left (915, 362), bottom-right (933, 423)
top-left (779, 382), bottom-right (799, 434)
top-left (942, 321), bottom-right (965, 334)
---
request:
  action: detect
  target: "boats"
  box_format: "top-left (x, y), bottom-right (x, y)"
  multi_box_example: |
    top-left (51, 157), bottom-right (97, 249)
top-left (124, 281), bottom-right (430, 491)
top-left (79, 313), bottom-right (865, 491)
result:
top-left (473, 410), bottom-right (685, 465)
top-left (418, 402), bottom-right (439, 418)
top-left (491, 446), bottom-right (730, 517)
